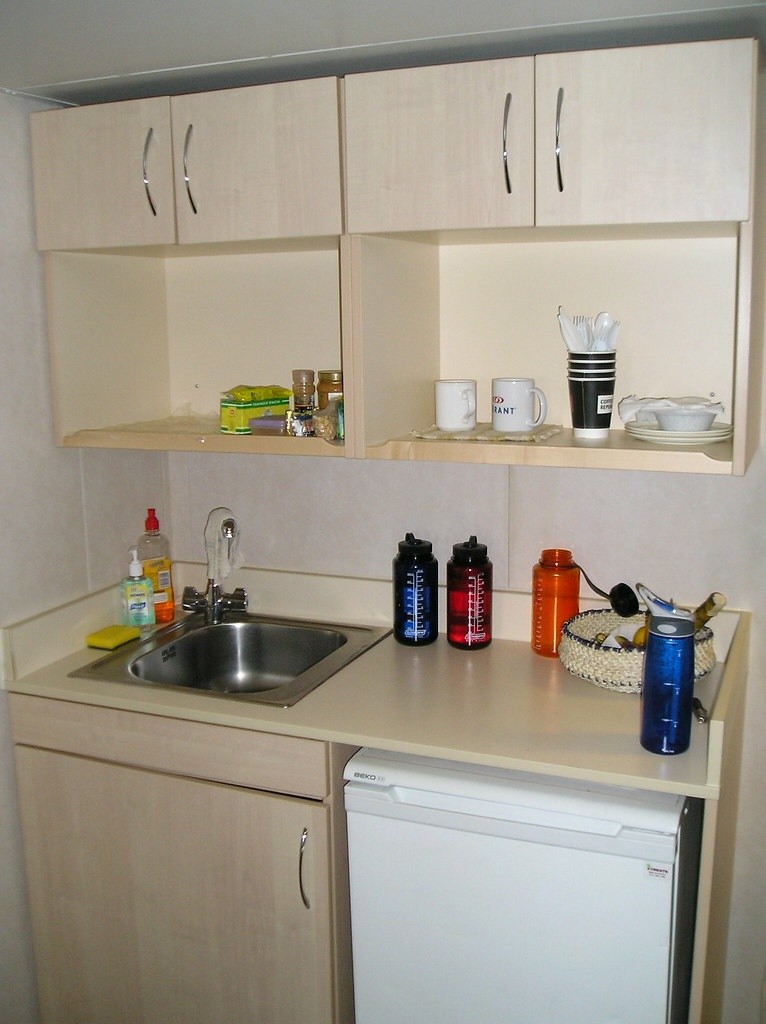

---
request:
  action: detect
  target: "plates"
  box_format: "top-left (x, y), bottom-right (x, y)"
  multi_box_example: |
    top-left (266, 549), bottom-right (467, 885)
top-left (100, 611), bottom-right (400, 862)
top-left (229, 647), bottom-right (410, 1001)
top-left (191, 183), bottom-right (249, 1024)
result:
top-left (624, 421), bottom-right (733, 445)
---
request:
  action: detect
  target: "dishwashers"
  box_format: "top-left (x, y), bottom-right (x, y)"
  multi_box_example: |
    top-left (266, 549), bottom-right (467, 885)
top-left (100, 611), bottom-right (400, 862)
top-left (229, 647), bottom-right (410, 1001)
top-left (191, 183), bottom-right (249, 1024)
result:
top-left (342, 748), bottom-right (701, 1024)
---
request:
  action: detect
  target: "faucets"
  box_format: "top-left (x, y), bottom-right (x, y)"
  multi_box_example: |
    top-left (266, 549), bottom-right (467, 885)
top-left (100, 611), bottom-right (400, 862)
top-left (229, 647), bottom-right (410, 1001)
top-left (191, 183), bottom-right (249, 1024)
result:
top-left (204, 506), bottom-right (243, 594)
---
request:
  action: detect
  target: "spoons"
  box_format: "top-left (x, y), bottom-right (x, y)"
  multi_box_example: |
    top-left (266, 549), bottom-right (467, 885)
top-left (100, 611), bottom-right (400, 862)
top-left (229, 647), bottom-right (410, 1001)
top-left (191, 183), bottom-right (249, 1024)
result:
top-left (596, 312), bottom-right (611, 329)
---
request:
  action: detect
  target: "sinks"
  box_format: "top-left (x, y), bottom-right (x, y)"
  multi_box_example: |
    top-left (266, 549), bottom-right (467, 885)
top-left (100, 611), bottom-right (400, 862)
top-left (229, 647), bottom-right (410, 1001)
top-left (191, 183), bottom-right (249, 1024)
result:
top-left (66, 605), bottom-right (394, 710)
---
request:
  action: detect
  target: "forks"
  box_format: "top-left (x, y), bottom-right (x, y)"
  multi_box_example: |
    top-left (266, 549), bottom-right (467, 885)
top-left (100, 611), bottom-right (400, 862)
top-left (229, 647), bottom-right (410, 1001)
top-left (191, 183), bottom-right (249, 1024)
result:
top-left (574, 316), bottom-right (620, 351)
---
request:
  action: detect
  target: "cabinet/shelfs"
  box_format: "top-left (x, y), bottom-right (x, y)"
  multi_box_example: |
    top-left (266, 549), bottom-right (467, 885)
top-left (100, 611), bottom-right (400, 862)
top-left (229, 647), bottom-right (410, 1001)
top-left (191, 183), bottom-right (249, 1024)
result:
top-left (342, 36), bottom-right (763, 479)
top-left (27, 75), bottom-right (357, 459)
top-left (6, 691), bottom-right (355, 1024)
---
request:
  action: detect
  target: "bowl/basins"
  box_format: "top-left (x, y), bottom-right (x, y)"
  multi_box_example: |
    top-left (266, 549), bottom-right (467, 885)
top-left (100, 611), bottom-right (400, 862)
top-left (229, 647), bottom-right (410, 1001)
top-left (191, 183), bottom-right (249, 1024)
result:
top-left (656, 413), bottom-right (716, 431)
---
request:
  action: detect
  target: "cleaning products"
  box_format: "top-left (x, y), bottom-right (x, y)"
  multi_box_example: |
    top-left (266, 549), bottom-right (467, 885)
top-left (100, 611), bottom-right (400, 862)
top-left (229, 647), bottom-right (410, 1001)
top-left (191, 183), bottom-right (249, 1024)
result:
top-left (137, 506), bottom-right (174, 622)
top-left (118, 543), bottom-right (155, 628)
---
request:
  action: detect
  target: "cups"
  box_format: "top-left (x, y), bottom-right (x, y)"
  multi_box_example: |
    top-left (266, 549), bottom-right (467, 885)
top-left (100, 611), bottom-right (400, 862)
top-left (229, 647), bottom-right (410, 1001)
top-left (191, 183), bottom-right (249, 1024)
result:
top-left (434, 380), bottom-right (477, 433)
top-left (567, 349), bottom-right (617, 439)
top-left (532, 549), bottom-right (639, 658)
top-left (636, 583), bottom-right (694, 756)
top-left (393, 533), bottom-right (438, 647)
top-left (492, 378), bottom-right (546, 431)
top-left (446, 536), bottom-right (493, 650)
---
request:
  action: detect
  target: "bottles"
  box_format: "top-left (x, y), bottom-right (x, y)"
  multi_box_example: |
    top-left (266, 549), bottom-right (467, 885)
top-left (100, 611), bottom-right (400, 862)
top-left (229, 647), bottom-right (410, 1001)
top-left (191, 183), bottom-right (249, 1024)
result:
top-left (291, 370), bottom-right (316, 412)
